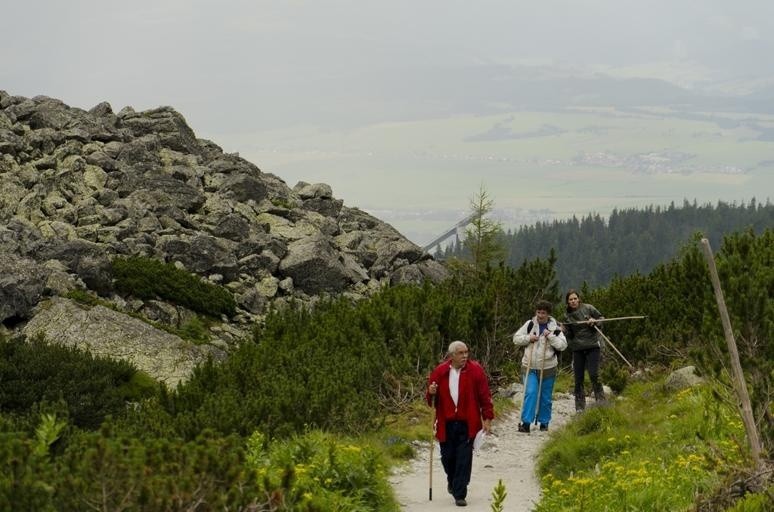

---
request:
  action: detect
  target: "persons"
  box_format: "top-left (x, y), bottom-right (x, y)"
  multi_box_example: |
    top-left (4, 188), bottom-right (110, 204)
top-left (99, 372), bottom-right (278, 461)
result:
top-left (557, 289), bottom-right (606, 414)
top-left (425, 341), bottom-right (495, 506)
top-left (511, 301), bottom-right (570, 431)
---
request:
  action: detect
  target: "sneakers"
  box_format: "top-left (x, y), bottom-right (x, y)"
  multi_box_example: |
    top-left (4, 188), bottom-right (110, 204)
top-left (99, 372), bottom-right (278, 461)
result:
top-left (518, 422), bottom-right (529, 431)
top-left (447, 482), bottom-right (468, 506)
top-left (540, 423), bottom-right (547, 430)
